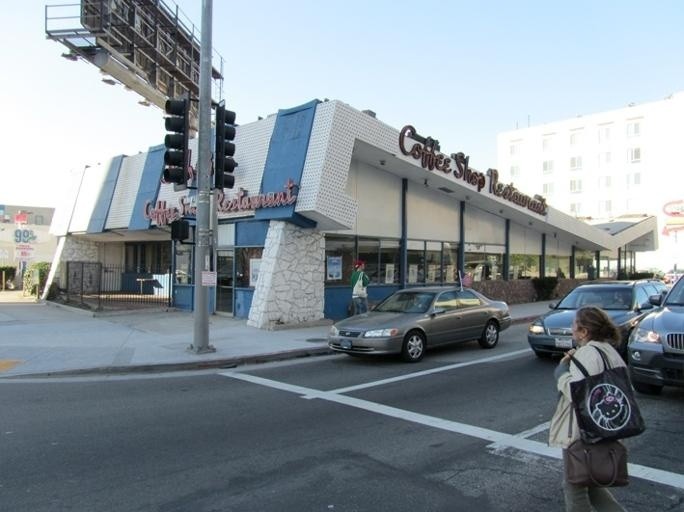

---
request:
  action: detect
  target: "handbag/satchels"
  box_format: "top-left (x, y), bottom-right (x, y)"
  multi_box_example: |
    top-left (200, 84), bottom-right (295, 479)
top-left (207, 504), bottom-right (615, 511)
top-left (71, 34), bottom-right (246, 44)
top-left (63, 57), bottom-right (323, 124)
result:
top-left (562, 437), bottom-right (629, 486)
top-left (352, 280), bottom-right (367, 298)
top-left (570, 366), bottom-right (645, 446)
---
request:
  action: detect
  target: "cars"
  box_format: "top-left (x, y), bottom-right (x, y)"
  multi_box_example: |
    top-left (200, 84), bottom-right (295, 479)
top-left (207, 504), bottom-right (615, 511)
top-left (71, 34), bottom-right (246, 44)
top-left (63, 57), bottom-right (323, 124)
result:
top-left (327, 286), bottom-right (512, 362)
top-left (527, 278), bottom-right (666, 360)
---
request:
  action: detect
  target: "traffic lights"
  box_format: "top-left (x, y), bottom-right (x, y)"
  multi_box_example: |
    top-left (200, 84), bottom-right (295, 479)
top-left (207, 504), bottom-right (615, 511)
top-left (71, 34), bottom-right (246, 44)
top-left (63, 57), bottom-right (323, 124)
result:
top-left (216, 104), bottom-right (237, 190)
top-left (164, 100), bottom-right (188, 184)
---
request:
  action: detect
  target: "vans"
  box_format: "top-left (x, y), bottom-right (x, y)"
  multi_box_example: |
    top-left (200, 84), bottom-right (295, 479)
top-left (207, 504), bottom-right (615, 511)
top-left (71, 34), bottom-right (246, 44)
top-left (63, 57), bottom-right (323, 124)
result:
top-left (626, 275), bottom-right (684, 396)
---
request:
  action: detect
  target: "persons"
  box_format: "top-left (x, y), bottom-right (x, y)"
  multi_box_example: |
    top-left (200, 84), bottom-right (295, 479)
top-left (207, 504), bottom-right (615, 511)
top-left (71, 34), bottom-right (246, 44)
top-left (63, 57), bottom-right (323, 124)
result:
top-left (587, 263), bottom-right (596, 280)
top-left (350, 261), bottom-right (370, 314)
top-left (619, 269), bottom-right (627, 280)
top-left (548, 306), bottom-right (628, 512)
top-left (557, 267), bottom-right (565, 282)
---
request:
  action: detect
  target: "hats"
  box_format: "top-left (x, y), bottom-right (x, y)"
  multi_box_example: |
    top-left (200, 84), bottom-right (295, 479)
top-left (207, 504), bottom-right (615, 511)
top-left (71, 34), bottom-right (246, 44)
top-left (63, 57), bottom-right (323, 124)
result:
top-left (354, 260), bottom-right (366, 270)
